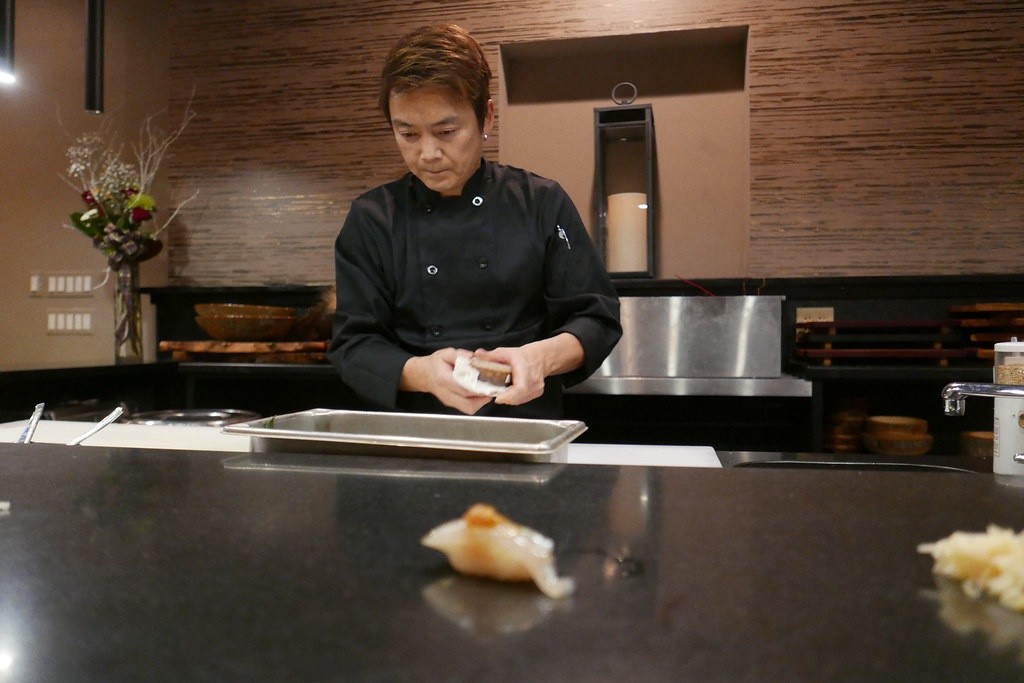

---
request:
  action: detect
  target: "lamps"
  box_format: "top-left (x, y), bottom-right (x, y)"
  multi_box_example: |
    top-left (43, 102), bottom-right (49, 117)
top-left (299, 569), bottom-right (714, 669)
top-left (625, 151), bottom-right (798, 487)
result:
top-left (0, 0), bottom-right (15, 84)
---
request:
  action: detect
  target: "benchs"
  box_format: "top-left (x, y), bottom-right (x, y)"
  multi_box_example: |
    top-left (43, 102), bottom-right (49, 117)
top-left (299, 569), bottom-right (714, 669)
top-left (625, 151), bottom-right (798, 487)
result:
top-left (789, 300), bottom-right (1024, 453)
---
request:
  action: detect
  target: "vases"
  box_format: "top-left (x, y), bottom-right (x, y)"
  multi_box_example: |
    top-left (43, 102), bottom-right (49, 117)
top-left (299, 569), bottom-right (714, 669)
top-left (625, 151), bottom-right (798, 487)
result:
top-left (112, 259), bottom-right (143, 367)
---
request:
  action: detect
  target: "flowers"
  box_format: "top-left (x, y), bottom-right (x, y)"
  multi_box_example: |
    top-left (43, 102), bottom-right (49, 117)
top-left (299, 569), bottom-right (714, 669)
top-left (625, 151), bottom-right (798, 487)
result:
top-left (55, 84), bottom-right (196, 271)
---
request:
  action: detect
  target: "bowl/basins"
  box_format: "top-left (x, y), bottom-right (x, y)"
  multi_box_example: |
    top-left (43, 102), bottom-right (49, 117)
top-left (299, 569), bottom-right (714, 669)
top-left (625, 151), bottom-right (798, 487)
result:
top-left (961, 432), bottom-right (993, 457)
top-left (824, 409), bottom-right (933, 455)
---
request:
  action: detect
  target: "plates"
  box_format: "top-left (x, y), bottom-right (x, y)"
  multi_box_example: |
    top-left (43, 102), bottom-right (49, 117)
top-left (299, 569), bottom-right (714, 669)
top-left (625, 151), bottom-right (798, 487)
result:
top-left (194, 302), bottom-right (321, 340)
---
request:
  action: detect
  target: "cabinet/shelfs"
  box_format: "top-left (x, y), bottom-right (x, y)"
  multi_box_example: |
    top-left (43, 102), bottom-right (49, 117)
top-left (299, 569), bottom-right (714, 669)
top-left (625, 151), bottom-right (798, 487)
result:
top-left (129, 288), bottom-right (344, 413)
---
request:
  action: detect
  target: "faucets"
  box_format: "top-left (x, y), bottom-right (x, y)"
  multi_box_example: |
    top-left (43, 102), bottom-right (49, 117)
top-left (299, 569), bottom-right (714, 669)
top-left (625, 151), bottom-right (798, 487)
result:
top-left (939, 380), bottom-right (1024, 418)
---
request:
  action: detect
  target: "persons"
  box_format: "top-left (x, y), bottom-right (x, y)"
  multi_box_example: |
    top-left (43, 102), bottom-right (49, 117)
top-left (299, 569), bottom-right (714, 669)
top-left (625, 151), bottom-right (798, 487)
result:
top-left (326, 23), bottom-right (623, 419)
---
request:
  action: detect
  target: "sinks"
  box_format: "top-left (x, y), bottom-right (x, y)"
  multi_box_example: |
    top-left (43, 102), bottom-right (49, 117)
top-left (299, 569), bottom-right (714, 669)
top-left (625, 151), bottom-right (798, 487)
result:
top-left (714, 449), bottom-right (996, 480)
